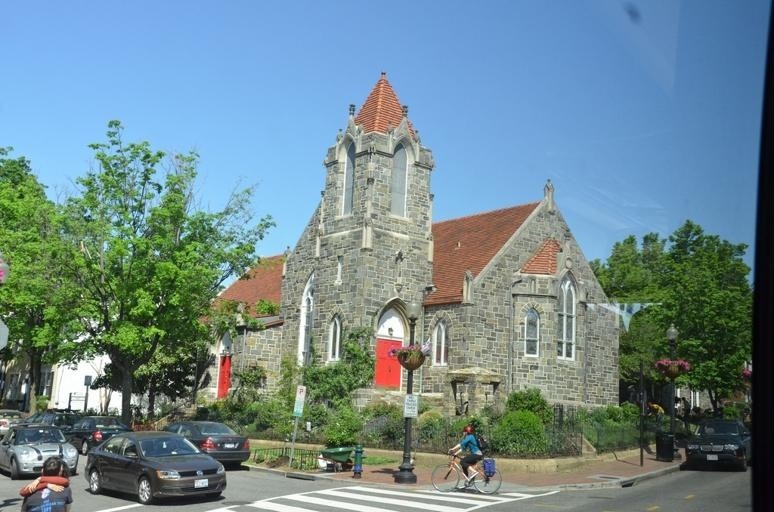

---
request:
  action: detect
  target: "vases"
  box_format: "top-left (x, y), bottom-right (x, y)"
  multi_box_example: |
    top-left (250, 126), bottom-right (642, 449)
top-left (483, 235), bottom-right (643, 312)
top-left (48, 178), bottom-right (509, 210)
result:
top-left (664, 365), bottom-right (683, 378)
top-left (397, 353), bottom-right (425, 370)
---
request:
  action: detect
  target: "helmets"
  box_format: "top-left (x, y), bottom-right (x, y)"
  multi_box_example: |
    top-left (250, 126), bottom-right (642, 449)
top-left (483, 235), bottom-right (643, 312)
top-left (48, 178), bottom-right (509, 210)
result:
top-left (463, 425), bottom-right (473, 434)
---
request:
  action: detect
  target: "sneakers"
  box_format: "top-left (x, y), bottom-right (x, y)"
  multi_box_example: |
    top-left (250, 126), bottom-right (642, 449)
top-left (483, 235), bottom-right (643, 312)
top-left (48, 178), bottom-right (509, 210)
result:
top-left (469, 469), bottom-right (479, 480)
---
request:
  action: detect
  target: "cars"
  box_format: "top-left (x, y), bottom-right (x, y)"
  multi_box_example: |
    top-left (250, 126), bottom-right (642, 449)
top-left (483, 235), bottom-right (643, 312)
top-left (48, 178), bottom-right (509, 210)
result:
top-left (685, 419), bottom-right (752, 472)
top-left (162, 420), bottom-right (251, 465)
top-left (84, 431), bottom-right (227, 504)
top-left (1, 408), bottom-right (135, 480)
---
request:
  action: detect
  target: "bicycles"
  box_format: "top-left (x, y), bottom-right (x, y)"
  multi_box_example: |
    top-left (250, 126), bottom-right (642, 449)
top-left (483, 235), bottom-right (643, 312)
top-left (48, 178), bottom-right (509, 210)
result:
top-left (431, 450), bottom-right (503, 495)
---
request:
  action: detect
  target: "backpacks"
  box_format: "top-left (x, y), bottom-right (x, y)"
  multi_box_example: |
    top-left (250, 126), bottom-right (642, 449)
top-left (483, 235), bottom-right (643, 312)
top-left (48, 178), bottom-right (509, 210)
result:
top-left (471, 434), bottom-right (488, 451)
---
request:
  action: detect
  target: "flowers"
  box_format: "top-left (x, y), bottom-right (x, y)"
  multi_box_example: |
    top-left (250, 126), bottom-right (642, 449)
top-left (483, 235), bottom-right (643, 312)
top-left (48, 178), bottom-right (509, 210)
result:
top-left (388, 344), bottom-right (424, 364)
top-left (654, 359), bottom-right (690, 376)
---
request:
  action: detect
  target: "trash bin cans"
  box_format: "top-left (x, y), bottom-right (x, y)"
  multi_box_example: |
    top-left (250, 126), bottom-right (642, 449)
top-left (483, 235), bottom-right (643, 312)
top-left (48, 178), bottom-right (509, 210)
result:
top-left (655, 430), bottom-right (675, 462)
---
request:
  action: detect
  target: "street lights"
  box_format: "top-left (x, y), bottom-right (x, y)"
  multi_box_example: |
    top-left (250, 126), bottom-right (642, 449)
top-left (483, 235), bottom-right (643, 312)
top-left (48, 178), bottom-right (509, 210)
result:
top-left (666, 323), bottom-right (682, 460)
top-left (394, 301), bottom-right (422, 484)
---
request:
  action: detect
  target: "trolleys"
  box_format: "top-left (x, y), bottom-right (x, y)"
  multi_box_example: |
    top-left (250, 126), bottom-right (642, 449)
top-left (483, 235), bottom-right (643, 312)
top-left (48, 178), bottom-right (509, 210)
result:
top-left (320, 447), bottom-right (365, 469)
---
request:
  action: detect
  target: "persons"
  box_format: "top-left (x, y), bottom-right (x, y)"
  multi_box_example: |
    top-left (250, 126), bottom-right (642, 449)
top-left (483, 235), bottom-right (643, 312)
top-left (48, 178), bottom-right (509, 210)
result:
top-left (22, 457), bottom-right (75, 512)
top-left (681, 397), bottom-right (690, 417)
top-left (646, 401), bottom-right (664, 430)
top-left (18, 461), bottom-right (71, 496)
top-left (448, 425), bottom-right (483, 489)
